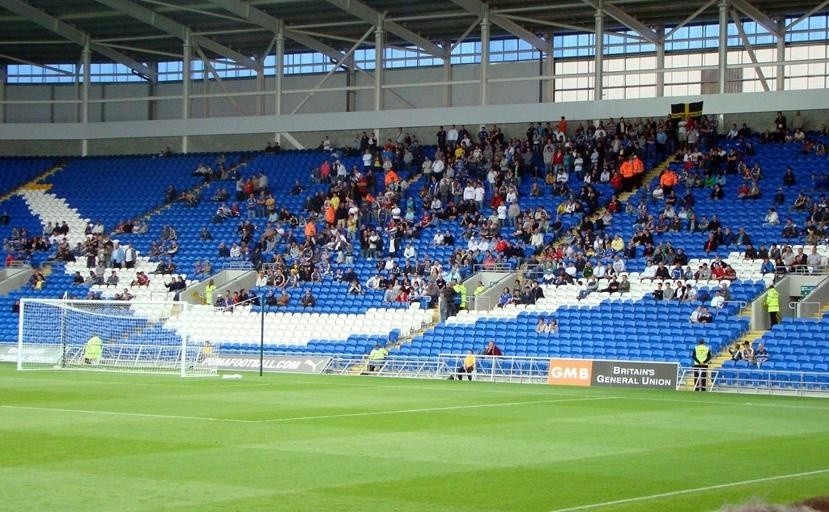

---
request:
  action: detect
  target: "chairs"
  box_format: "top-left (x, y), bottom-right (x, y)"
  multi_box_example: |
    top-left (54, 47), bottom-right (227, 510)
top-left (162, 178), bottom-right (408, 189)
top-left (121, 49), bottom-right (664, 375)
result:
top-left (0, 129), bottom-right (828, 396)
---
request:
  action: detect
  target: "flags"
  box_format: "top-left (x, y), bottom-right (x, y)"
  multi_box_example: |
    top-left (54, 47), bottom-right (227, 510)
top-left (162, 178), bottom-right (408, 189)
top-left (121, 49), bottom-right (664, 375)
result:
top-left (671, 101), bottom-right (703, 128)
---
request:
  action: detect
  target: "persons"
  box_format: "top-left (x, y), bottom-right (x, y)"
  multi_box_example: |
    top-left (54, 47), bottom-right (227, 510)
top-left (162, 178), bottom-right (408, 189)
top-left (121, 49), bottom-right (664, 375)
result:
top-left (84, 333), bottom-right (101, 364)
top-left (728, 341), bottom-right (767, 361)
top-left (197, 340), bottom-right (212, 363)
top-left (481, 342), bottom-right (501, 359)
top-left (2, 212), bottom-right (150, 312)
top-left (369, 345), bottom-right (388, 371)
top-left (693, 339), bottom-right (711, 391)
top-left (152, 110), bottom-right (829, 333)
top-left (458, 351), bottom-right (476, 380)
